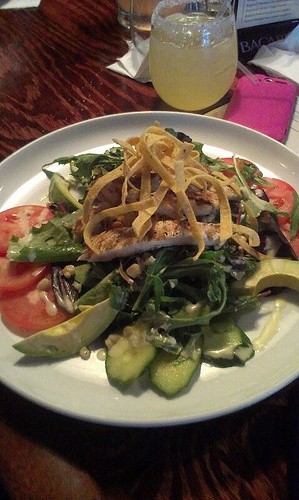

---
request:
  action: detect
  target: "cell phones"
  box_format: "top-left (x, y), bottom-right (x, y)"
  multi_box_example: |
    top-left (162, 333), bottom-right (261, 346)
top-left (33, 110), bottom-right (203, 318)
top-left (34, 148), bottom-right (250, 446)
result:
top-left (223, 74), bottom-right (299, 142)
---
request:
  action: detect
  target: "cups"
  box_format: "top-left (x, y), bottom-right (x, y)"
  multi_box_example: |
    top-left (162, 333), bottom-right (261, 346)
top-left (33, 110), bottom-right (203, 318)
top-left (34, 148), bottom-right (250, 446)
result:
top-left (148, 1), bottom-right (238, 112)
top-left (118, 0), bottom-right (186, 31)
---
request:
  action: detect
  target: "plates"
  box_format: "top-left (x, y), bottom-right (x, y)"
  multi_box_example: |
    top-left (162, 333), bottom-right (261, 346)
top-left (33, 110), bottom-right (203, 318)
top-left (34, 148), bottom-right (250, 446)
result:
top-left (1, 111), bottom-right (299, 428)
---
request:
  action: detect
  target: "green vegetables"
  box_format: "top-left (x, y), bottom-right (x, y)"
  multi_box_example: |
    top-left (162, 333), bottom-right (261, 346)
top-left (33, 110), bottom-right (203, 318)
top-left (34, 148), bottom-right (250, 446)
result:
top-left (232, 153), bottom-right (299, 239)
top-left (8, 127), bottom-right (252, 338)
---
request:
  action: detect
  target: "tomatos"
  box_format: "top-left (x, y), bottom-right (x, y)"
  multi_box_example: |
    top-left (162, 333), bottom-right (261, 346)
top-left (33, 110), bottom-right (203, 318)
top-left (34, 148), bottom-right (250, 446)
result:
top-left (0, 205), bottom-right (71, 332)
top-left (218, 159), bottom-right (299, 259)
